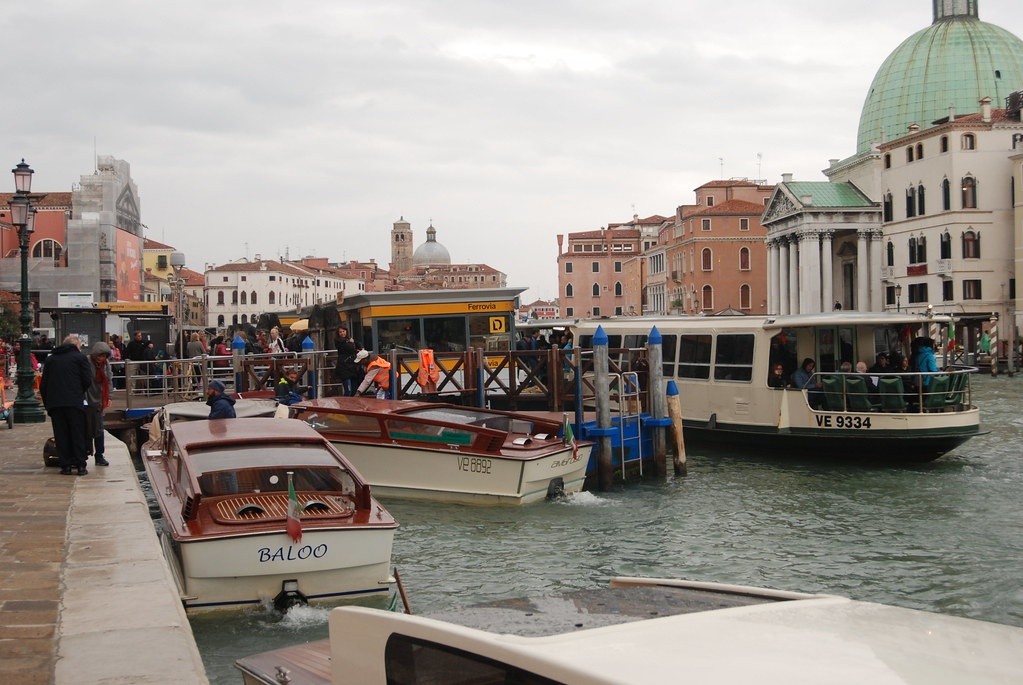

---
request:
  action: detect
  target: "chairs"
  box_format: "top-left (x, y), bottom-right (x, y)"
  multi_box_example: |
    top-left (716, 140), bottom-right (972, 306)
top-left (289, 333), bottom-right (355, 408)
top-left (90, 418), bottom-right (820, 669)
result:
top-left (822, 373), bottom-right (967, 413)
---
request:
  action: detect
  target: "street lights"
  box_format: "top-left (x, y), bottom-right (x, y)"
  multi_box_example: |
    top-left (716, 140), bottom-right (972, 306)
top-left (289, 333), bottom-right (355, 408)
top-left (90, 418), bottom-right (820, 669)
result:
top-left (7, 157), bottom-right (48, 422)
top-left (167, 250), bottom-right (190, 399)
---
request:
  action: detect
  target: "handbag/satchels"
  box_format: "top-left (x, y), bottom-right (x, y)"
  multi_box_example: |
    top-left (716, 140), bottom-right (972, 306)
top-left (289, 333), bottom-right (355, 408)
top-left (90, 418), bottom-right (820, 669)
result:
top-left (43, 437), bottom-right (60, 467)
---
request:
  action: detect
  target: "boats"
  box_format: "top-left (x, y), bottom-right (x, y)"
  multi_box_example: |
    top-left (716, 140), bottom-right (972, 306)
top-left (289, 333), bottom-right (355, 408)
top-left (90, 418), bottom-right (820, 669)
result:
top-left (229, 570), bottom-right (1021, 684)
top-left (287, 392), bottom-right (596, 507)
top-left (509, 308), bottom-right (993, 461)
top-left (140, 396), bottom-right (400, 615)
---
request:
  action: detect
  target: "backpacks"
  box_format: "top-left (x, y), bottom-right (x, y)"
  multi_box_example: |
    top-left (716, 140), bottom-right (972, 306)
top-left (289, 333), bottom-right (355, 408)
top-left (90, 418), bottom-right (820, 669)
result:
top-left (913, 365), bottom-right (924, 387)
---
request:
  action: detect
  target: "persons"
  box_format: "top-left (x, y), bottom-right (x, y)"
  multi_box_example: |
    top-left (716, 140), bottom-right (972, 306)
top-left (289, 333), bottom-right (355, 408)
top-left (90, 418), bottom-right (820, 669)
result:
top-left (0, 326), bottom-right (399, 475)
top-left (518, 328), bottom-right (574, 385)
top-left (770, 337), bottom-right (942, 410)
top-left (980, 329), bottom-right (992, 357)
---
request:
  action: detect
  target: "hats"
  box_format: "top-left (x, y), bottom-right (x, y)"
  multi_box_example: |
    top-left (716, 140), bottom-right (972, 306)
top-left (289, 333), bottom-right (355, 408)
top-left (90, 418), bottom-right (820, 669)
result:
top-left (354, 350), bottom-right (369, 363)
top-left (208, 379), bottom-right (225, 393)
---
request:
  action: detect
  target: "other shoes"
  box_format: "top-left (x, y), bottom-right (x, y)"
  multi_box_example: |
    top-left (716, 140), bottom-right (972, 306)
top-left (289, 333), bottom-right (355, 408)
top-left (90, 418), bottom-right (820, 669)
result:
top-left (60, 466), bottom-right (72, 475)
top-left (78, 466), bottom-right (88, 475)
top-left (94, 454), bottom-right (109, 466)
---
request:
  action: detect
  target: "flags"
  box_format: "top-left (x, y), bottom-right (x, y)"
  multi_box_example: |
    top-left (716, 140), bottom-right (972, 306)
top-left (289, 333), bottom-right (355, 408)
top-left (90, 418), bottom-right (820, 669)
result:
top-left (948, 322), bottom-right (956, 352)
top-left (286, 479), bottom-right (302, 543)
